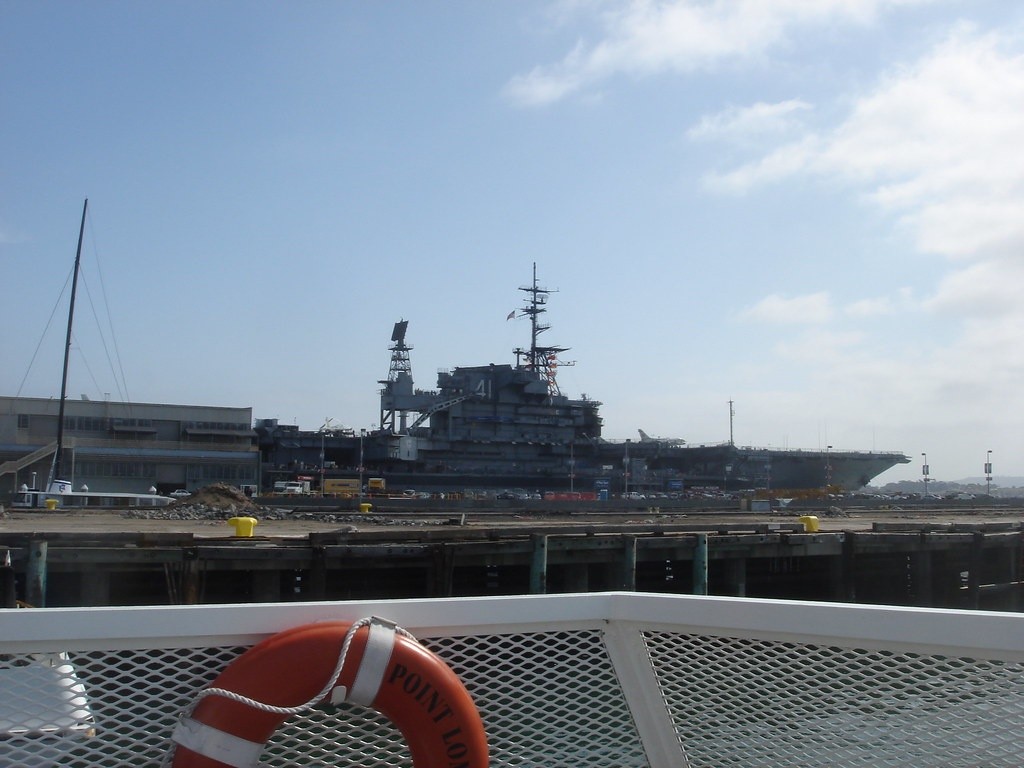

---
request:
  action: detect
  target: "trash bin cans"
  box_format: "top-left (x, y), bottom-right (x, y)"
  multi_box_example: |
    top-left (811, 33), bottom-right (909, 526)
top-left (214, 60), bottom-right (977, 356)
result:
top-left (740, 498), bottom-right (747, 511)
top-left (600, 489), bottom-right (608, 500)
top-left (751, 499), bottom-right (770, 511)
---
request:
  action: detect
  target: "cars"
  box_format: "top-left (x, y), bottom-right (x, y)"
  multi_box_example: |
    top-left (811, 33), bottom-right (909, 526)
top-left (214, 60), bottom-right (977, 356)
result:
top-left (497, 492), bottom-right (582, 499)
top-left (403, 490), bottom-right (415, 497)
top-left (168, 489), bottom-right (191, 498)
top-left (646, 491), bottom-right (731, 498)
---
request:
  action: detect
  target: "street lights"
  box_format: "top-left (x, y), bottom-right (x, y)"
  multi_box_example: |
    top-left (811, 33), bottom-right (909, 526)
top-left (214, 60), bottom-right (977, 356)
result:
top-left (570, 441), bottom-right (574, 493)
top-left (922, 453), bottom-right (928, 497)
top-left (825, 446), bottom-right (833, 493)
top-left (987, 451), bottom-right (993, 494)
top-left (360, 428), bottom-right (366, 499)
top-left (623, 438), bottom-right (631, 491)
top-left (321, 432), bottom-right (326, 497)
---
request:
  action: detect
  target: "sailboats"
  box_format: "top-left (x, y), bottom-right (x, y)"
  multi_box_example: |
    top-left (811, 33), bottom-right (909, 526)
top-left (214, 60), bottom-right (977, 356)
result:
top-left (1, 199), bottom-right (176, 509)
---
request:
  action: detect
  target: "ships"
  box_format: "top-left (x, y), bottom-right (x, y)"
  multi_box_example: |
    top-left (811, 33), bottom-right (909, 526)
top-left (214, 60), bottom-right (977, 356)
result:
top-left (251, 260), bottom-right (912, 495)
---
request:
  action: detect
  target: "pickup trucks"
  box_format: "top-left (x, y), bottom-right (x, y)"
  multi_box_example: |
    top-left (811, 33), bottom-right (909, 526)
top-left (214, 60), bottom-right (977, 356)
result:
top-left (621, 491), bottom-right (646, 500)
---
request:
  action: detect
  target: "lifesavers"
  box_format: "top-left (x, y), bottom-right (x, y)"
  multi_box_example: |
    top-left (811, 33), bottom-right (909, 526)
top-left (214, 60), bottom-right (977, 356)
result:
top-left (168, 621), bottom-right (489, 768)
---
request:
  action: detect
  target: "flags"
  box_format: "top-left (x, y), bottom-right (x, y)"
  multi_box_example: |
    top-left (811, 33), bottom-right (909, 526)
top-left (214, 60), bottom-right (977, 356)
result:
top-left (507, 311), bottom-right (515, 321)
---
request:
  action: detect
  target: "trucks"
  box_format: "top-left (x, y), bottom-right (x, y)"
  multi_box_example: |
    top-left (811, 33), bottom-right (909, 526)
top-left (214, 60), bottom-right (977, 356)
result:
top-left (272, 481), bottom-right (287, 495)
top-left (369, 478), bottom-right (385, 493)
top-left (286, 482), bottom-right (318, 495)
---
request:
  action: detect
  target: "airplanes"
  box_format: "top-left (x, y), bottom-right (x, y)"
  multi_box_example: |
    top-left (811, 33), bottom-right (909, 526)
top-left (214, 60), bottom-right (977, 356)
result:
top-left (636, 429), bottom-right (686, 446)
